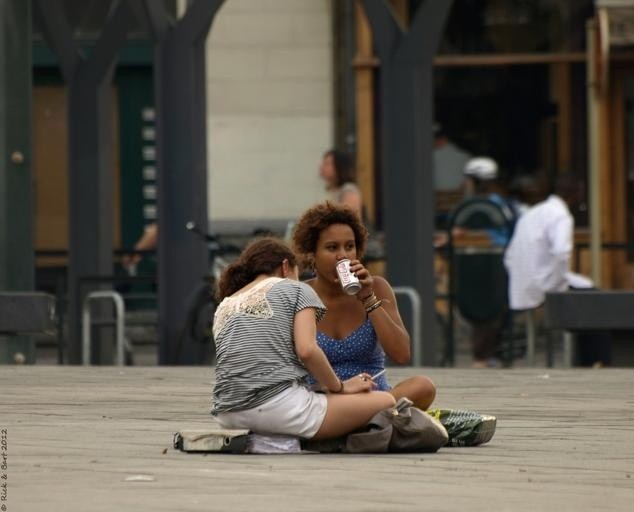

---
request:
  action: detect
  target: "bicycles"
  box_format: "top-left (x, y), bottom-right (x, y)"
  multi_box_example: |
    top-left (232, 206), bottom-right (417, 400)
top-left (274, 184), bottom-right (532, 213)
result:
top-left (181, 222), bottom-right (272, 366)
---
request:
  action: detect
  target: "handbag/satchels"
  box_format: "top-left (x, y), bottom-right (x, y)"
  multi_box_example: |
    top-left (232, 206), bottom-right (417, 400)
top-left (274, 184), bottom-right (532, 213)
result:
top-left (346, 398), bottom-right (448, 455)
top-left (433, 409), bottom-right (497, 447)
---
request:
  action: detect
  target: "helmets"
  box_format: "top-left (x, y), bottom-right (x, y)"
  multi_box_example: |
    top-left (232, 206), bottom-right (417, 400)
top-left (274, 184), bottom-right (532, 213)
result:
top-left (463, 157), bottom-right (498, 181)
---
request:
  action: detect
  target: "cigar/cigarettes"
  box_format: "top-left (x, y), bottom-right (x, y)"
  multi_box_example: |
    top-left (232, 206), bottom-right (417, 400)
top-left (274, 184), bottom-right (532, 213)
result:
top-left (368, 368), bottom-right (386, 381)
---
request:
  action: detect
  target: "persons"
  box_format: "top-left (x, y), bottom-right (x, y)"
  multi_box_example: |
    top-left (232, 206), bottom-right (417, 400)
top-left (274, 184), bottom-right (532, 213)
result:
top-left (503, 168), bottom-right (616, 370)
top-left (316, 148), bottom-right (364, 224)
top-left (430, 122), bottom-right (475, 192)
top-left (288, 198), bottom-right (438, 413)
top-left (211, 235), bottom-right (397, 445)
top-left (434, 155), bottom-right (513, 360)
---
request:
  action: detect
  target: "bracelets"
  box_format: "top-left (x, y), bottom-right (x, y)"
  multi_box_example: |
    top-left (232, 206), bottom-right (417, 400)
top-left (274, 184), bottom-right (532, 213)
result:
top-left (358, 288), bottom-right (383, 313)
top-left (326, 379), bottom-right (344, 394)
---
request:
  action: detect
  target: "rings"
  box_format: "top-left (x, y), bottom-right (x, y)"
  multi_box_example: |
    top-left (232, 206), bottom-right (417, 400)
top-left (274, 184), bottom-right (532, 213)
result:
top-left (360, 376), bottom-right (367, 382)
top-left (357, 373), bottom-right (363, 378)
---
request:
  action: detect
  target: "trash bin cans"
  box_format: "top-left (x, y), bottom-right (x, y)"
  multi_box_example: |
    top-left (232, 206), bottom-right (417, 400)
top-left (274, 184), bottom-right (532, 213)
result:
top-left (440, 193), bottom-right (519, 370)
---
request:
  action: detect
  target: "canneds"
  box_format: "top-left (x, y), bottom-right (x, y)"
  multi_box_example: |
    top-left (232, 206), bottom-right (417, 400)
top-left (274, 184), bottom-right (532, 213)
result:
top-left (336, 258), bottom-right (362, 296)
